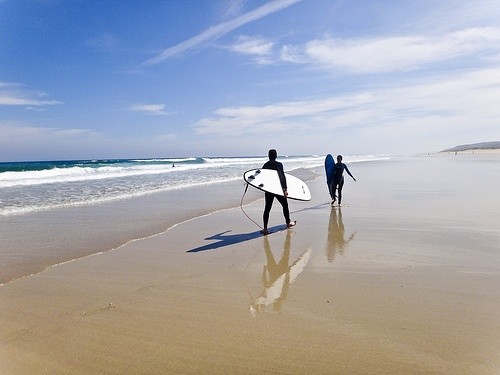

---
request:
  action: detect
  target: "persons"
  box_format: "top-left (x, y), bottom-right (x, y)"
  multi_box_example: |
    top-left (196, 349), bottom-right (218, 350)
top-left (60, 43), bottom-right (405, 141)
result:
top-left (328, 155), bottom-right (356, 207)
top-left (262, 149), bottom-right (297, 235)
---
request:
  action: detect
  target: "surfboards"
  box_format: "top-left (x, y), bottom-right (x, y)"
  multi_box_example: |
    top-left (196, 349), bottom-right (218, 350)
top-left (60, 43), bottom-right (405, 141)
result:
top-left (243, 167), bottom-right (312, 202)
top-left (324, 154), bottom-right (337, 200)
